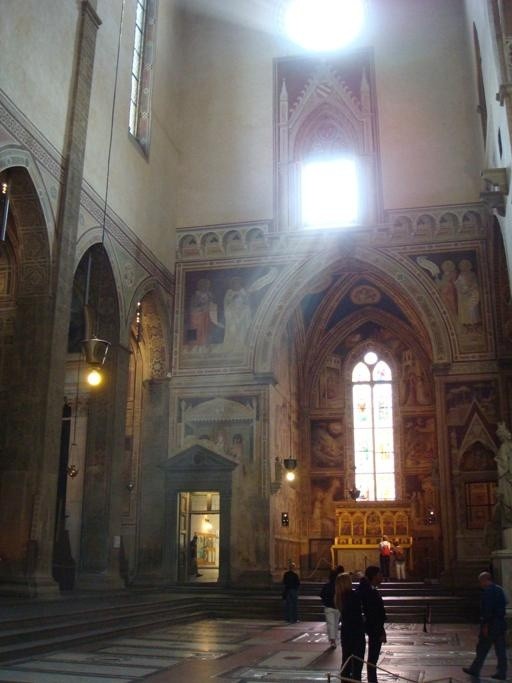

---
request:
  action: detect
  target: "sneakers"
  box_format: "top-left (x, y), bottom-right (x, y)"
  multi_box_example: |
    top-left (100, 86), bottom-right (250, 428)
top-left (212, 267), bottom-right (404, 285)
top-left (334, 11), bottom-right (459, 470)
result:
top-left (491, 673), bottom-right (506, 680)
top-left (332, 643), bottom-right (336, 648)
top-left (463, 667), bottom-right (481, 678)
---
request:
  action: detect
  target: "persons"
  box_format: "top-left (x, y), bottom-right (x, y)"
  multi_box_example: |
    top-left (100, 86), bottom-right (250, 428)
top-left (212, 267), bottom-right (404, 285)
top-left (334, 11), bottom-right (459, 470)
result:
top-left (439, 259), bottom-right (457, 314)
top-left (189, 534), bottom-right (203, 577)
top-left (333, 573), bottom-right (367, 683)
top-left (318, 570), bottom-right (342, 649)
top-left (227, 433), bottom-right (244, 458)
top-left (190, 277), bottom-right (218, 344)
top-left (357, 565), bottom-right (387, 682)
top-left (389, 538), bottom-right (407, 581)
top-left (453, 259), bottom-right (481, 325)
top-left (494, 422), bottom-right (511, 522)
top-left (378, 534), bottom-right (391, 581)
top-left (221, 277), bottom-right (252, 349)
top-left (461, 570), bottom-right (508, 680)
top-left (282, 561), bottom-right (301, 624)
